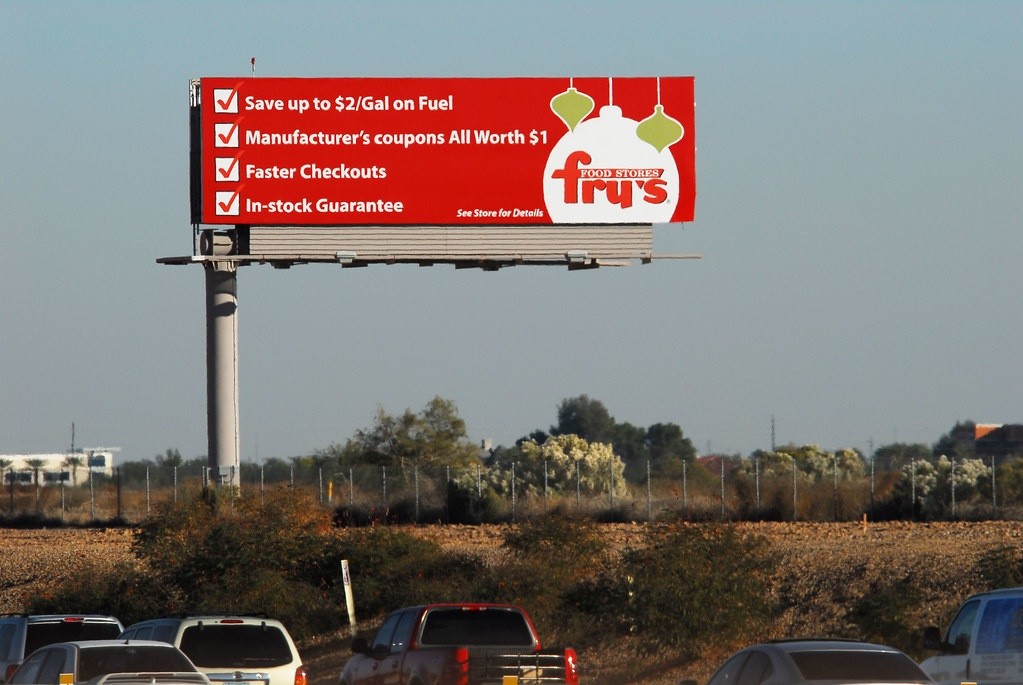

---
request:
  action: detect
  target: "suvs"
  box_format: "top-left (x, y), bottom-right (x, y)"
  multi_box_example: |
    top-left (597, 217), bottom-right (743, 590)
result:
top-left (115, 612), bottom-right (307, 685)
top-left (1, 613), bottom-right (125, 685)
top-left (917, 588), bottom-right (1023, 685)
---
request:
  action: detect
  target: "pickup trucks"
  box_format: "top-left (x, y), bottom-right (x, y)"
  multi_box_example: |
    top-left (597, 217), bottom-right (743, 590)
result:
top-left (337, 604), bottom-right (580, 685)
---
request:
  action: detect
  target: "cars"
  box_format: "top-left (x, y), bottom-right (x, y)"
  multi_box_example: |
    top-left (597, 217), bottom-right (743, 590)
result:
top-left (678, 637), bottom-right (940, 685)
top-left (4, 639), bottom-right (217, 685)
